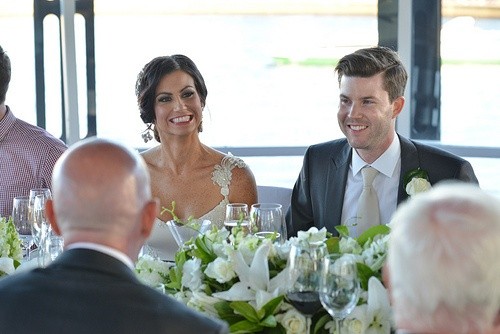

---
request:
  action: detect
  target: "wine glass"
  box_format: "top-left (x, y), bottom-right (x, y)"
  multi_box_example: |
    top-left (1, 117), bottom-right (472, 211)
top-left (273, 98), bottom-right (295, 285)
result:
top-left (284, 243), bottom-right (330, 334)
top-left (319, 253), bottom-right (359, 334)
top-left (11, 188), bottom-right (63, 267)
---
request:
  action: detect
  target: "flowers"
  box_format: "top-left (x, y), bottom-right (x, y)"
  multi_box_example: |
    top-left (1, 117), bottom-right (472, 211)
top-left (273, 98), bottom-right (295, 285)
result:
top-left (138, 206), bottom-right (392, 334)
top-left (404, 168), bottom-right (436, 202)
top-left (0, 216), bottom-right (24, 275)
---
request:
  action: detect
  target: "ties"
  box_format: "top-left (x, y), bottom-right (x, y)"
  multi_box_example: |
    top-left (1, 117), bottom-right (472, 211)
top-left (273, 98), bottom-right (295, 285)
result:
top-left (355, 167), bottom-right (381, 234)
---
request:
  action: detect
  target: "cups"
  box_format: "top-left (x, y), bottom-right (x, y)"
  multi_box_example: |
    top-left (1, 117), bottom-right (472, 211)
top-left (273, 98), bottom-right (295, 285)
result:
top-left (224, 203), bottom-right (248, 244)
top-left (254, 231), bottom-right (281, 245)
top-left (248, 203), bottom-right (287, 247)
top-left (166, 219), bottom-right (211, 250)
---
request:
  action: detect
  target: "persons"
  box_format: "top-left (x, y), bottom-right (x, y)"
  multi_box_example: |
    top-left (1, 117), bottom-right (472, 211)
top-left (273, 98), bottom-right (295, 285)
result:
top-left (381, 178), bottom-right (500, 334)
top-left (136, 54), bottom-right (258, 266)
top-left (0, 138), bottom-right (228, 334)
top-left (0, 45), bottom-right (68, 222)
top-left (279, 46), bottom-right (478, 244)
